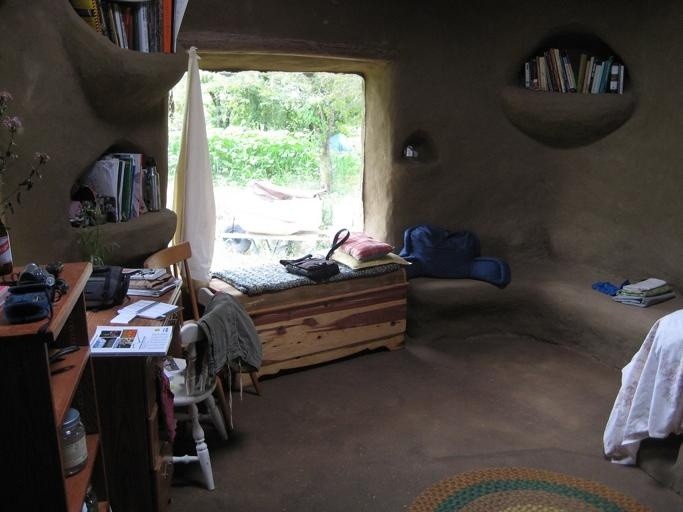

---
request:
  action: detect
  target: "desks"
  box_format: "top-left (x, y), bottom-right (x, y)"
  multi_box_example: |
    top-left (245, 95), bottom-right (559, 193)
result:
top-left (208, 268), bottom-right (410, 390)
top-left (86, 280), bottom-right (184, 512)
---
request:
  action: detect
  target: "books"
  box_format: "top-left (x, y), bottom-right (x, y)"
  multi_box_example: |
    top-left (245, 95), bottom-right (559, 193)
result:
top-left (88, 325), bottom-right (173, 356)
top-left (66, 1), bottom-right (191, 54)
top-left (114, 268), bottom-right (181, 298)
top-left (519, 46), bottom-right (626, 97)
top-left (80, 150), bottom-right (162, 223)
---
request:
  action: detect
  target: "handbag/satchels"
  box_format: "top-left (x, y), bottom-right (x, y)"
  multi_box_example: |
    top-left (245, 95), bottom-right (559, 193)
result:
top-left (280, 254), bottom-right (340, 281)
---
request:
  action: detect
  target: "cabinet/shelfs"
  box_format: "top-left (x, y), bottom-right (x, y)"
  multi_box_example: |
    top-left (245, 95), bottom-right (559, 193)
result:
top-left (0, 262), bottom-right (111, 512)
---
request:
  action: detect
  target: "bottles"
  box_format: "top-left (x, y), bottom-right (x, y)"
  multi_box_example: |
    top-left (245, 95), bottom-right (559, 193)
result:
top-left (0, 220), bottom-right (13, 275)
top-left (147, 167), bottom-right (161, 212)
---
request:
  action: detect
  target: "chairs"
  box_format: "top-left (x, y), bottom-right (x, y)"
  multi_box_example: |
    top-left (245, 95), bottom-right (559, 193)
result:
top-left (172, 324), bottom-right (228, 491)
top-left (144, 242), bottom-right (262, 440)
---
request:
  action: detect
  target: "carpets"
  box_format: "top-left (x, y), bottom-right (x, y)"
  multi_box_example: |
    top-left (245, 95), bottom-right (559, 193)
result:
top-left (409, 467), bottom-right (653, 512)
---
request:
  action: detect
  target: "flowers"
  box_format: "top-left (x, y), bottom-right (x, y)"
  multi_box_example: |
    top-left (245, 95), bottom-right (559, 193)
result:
top-left (0, 93), bottom-right (49, 226)
